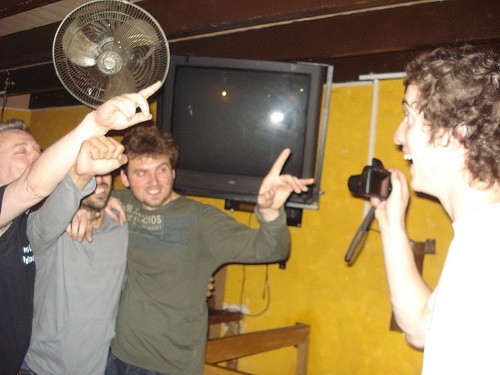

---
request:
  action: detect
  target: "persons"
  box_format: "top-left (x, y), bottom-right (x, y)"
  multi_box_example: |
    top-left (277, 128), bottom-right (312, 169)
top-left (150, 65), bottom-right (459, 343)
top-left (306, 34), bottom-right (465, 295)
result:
top-left (0, 80), bottom-right (315, 374)
top-left (368, 40), bottom-right (500, 372)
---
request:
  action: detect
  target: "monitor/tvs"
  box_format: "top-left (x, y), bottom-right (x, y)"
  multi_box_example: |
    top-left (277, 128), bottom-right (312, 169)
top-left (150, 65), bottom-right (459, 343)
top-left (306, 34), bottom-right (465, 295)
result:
top-left (155, 54), bottom-right (327, 205)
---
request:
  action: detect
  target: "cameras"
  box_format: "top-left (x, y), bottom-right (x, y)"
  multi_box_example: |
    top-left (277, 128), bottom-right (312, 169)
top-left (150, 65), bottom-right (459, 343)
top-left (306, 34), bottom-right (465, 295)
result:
top-left (347, 158), bottom-right (391, 201)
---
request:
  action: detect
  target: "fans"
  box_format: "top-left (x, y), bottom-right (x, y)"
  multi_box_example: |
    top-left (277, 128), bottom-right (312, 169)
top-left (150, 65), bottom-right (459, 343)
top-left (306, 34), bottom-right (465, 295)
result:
top-left (52, 0), bottom-right (171, 133)
